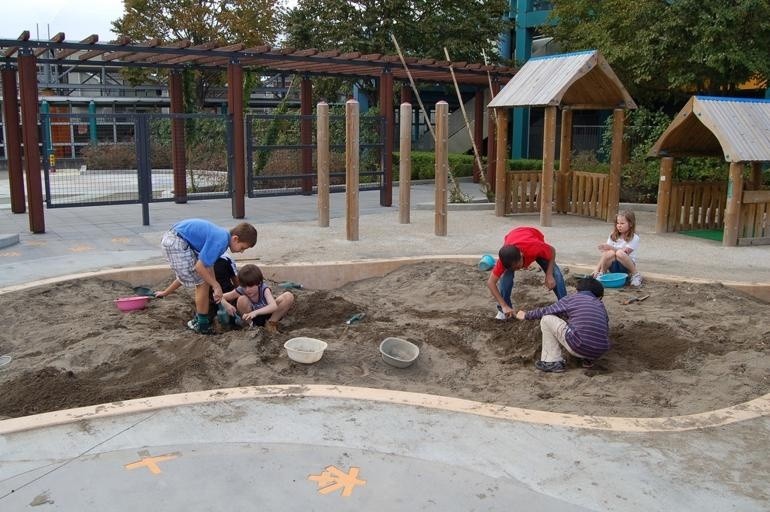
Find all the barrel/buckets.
[478,254,496,271]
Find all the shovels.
[623,293,650,305]
[232,311,249,328]
[346,312,366,325]
[574,273,593,279]
[133,286,163,299]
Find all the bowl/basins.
[596,272,629,291]
[283,336,329,364]
[380,337,420,367]
[113,295,149,312]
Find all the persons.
[153,218,295,335]
[516,277,611,373]
[593,208,643,287]
[487,226,568,320]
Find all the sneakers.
[496,311,507,318]
[187,318,216,335]
[581,359,593,368]
[535,360,566,373]
[630,273,643,286]
